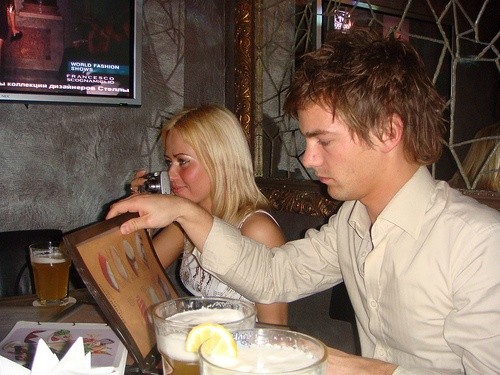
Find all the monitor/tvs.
[0,0,142,107]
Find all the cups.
[151,295,257,375]
[29,241,72,304]
[199,328,327,375]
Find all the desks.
[0,287,90,374]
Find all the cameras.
[138,170,170,195]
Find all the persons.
[130,105,288,327]
[105,26,500,375]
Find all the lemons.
[185,323,238,358]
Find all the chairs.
[0,229,66,296]
[249,185,348,326]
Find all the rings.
[130,186,137,193]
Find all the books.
[45,212,180,375]
